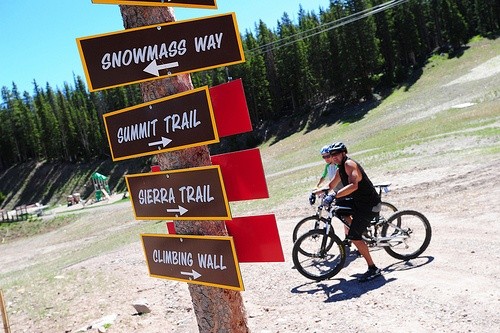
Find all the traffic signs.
[102,85,221,162]
[124,164,233,221]
[139,232,245,291]
[75,11,246,93]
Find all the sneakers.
[358,265,384,280]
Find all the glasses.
[322,154,331,159]
[331,152,342,156]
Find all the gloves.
[308,193,317,205]
[323,192,336,205]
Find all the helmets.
[328,142,348,154]
[320,145,332,154]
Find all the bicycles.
[292,183,402,258]
[292,191,432,281]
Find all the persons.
[312,145,351,248]
[309,141,381,281]
